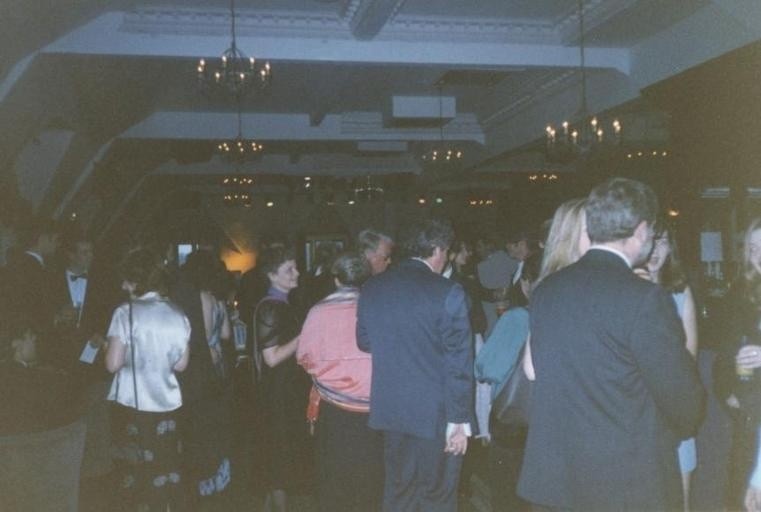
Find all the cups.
[496,299,508,319]
[735,335,754,382]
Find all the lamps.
[194,2,273,98]
[542,1,623,155]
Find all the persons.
[1,178,760,511]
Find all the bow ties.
[69,272,87,282]
